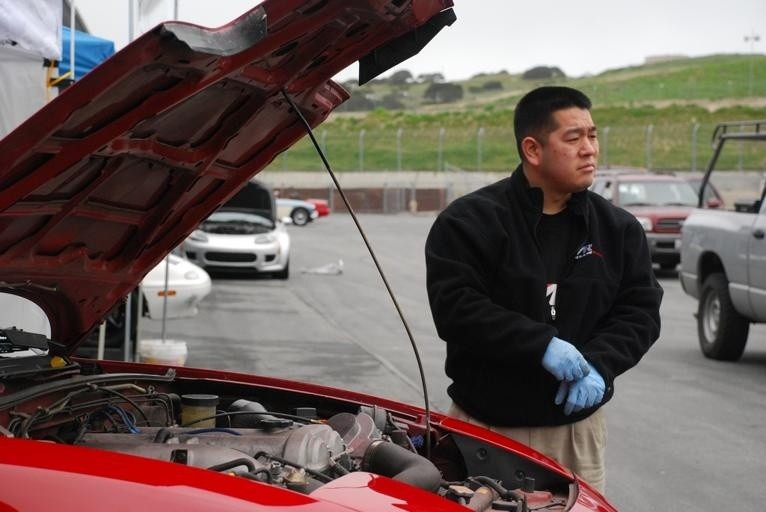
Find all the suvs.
[591,167,724,271]
[676,120,765,360]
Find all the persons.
[424,85,664,500]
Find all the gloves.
[555,361,606,415]
[541,335,591,382]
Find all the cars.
[141,180,329,318]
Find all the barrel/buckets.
[140,337,188,365]
